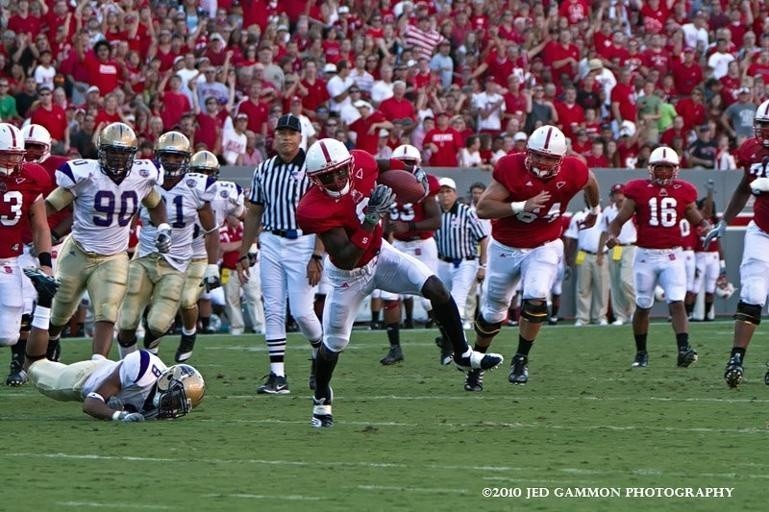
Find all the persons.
[0,0,769,430]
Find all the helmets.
[0,123,52,182]
[305,138,355,198]
[647,147,680,188]
[753,101,769,149]
[526,126,567,180]
[157,365,205,419]
[156,132,221,181]
[98,123,137,178]
[391,145,422,167]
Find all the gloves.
[199,277,218,293]
[118,411,145,421]
[154,231,172,254]
[365,185,397,224]
[417,170,430,203]
[702,221,726,249]
[750,178,769,195]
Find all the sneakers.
[311,385,334,428]
[381,346,403,364]
[257,372,289,394]
[678,347,697,367]
[144,328,161,355]
[508,355,528,383]
[632,350,648,367]
[46,339,61,363]
[7,361,29,386]
[175,331,196,362]
[23,266,60,294]
[310,350,317,389]
[434,336,503,392]
[724,364,743,388]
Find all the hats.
[438,177,456,190]
[275,116,302,132]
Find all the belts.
[272,228,314,237]
[438,252,474,262]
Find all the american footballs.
[378,169,426,203]
